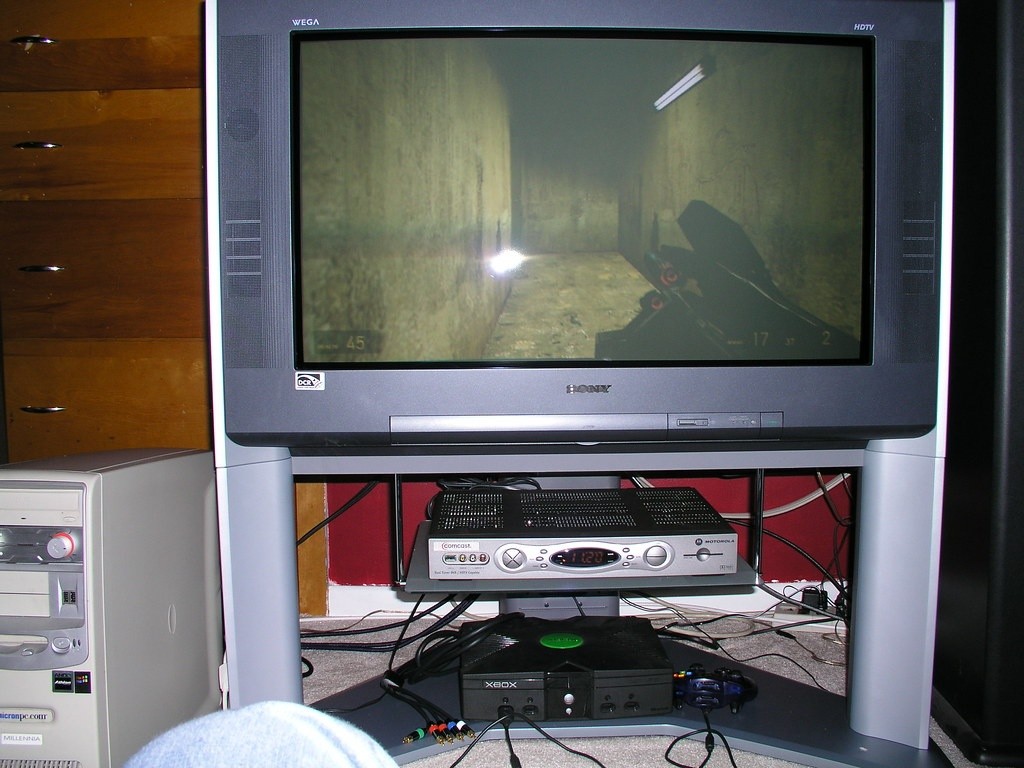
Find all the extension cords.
[772,603,849,637]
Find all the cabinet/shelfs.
[0,0,330,618]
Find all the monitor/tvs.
[205,0,946,460]
